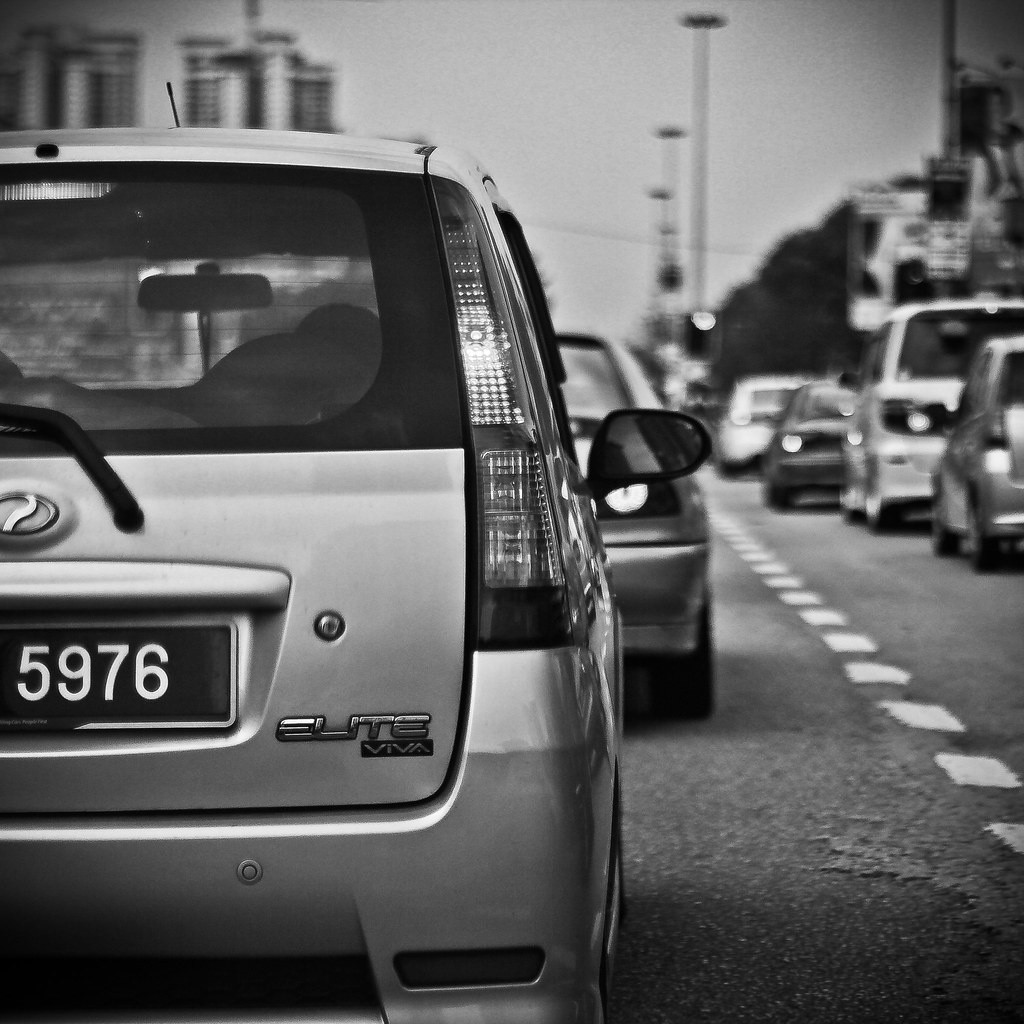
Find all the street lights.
[674,13,731,351]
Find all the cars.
[916,336,1024,570]
[718,374,817,476]
[556,328,713,723]
[764,383,851,507]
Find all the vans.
[838,296,1024,532]
[0,124,626,1024]
